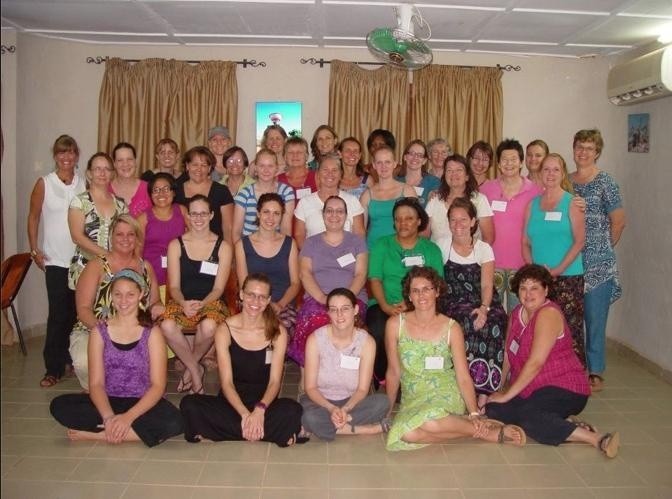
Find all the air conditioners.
[607,45,672,106]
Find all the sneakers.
[40,374,61,387]
[588,375,602,392]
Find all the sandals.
[472,419,526,447]
[567,414,620,459]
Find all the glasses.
[243,289,267,303]
[226,158,244,165]
[190,211,210,217]
[152,187,170,193]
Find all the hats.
[207,126,230,140]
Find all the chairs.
[0,252,33,355]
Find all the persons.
[180,272,311,448]
[49,264,187,448]
[385,265,527,451]
[299,285,393,440]
[479,264,620,459]
[25,122,626,415]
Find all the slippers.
[176,364,207,396]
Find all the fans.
[366,4,433,71]
